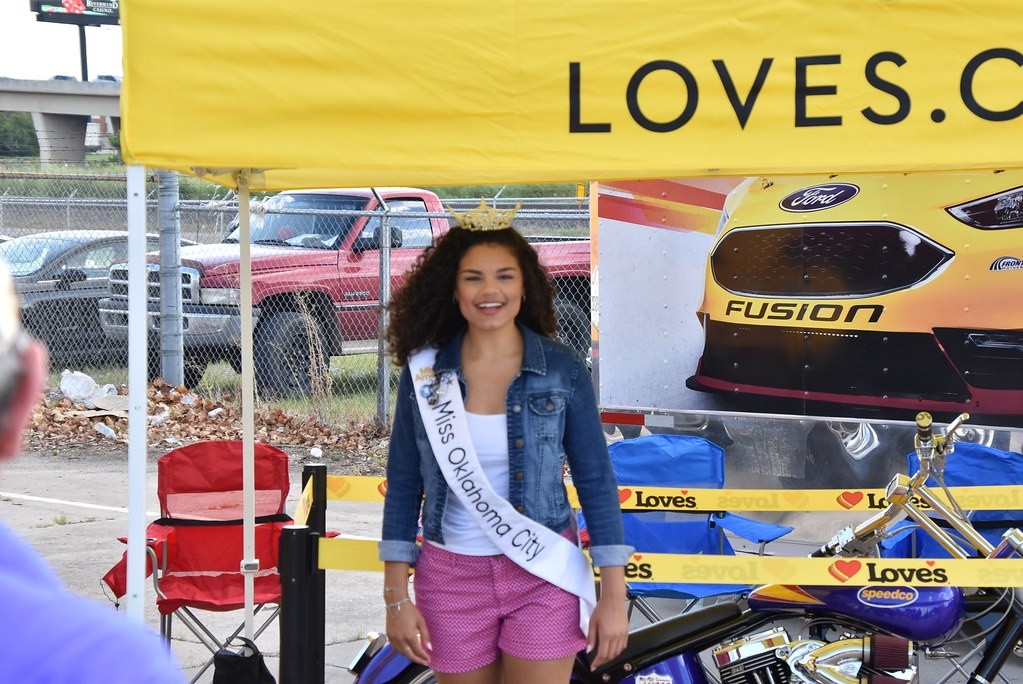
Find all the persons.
[0,259,185,683]
[378,196,634,684]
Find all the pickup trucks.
[98,188,589,396]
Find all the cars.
[684,171,1023,429]
[2,230,208,371]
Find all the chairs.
[100,438,295,683]
[371,226,403,248]
[871,441,1023,684]
[574,435,794,630]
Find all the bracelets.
[384,588,396,591]
[385,597,410,618]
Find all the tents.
[118,0,1023,630]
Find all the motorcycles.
[356,411,1023,684]
